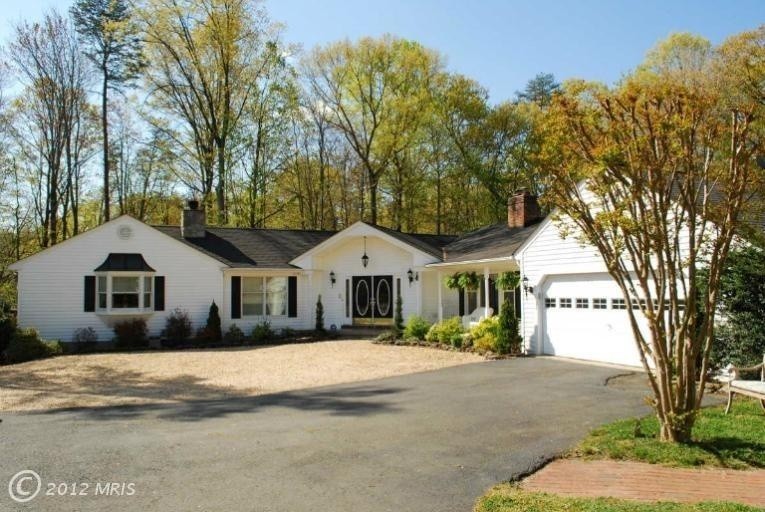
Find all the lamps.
[329,272,335,286]
[408,269,419,285]
[361,236,369,266]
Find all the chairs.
[724,363,765,414]
[462,306,494,329]
